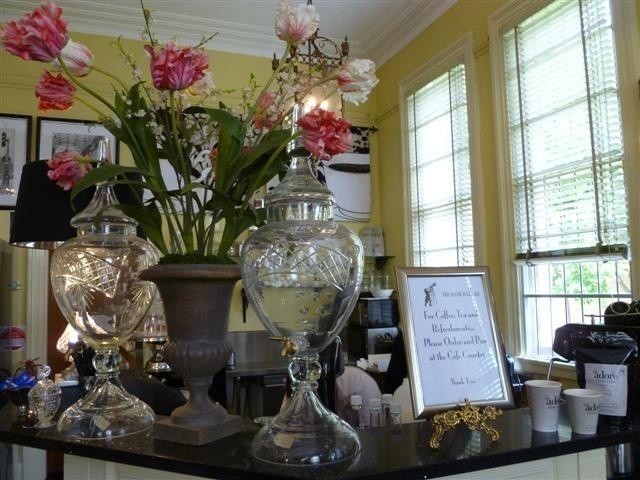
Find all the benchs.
[225,328,294,407]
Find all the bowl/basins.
[370,287,393,298]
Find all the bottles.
[348,393,403,429]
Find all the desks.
[216,360,334,419]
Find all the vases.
[135,262,253,447]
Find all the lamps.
[302,0,329,115]
[55,322,78,381]
[241,264,265,324]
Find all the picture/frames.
[35,115,119,166]
[1,113,32,210]
[393,265,516,420]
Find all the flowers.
[0,0,382,265]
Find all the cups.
[563,388,603,436]
[524,379,562,433]
